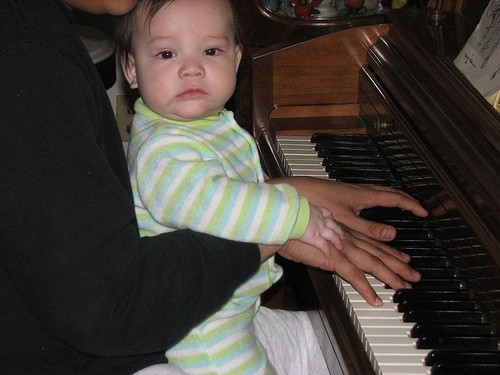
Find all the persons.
[0,0,428,375]
[117,0,348,374]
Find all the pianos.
[251,0,500,375]
